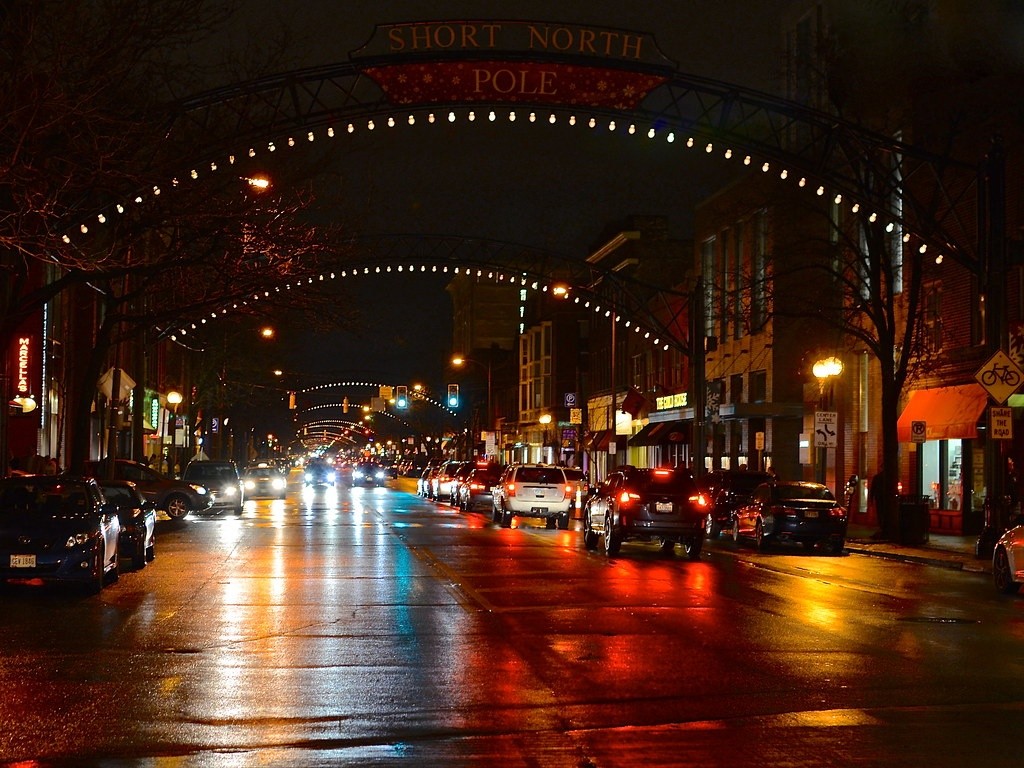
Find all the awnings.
[581,429,621,451]
[897,383,987,443]
[628,421,690,446]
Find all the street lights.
[538,414,552,464]
[324,357,492,453]
[104,177,282,479]
[551,285,617,469]
[812,356,845,485]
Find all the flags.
[621,389,646,417]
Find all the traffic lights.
[450,386,457,406]
[398,387,406,408]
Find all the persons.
[739,463,747,470]
[767,467,780,482]
[9,445,62,475]
[679,461,694,476]
[141,453,172,475]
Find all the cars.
[417,460,503,511]
[492,463,571,529]
[182,459,244,516]
[562,467,588,506]
[0,476,121,594]
[582,464,710,557]
[399,459,426,476]
[58,458,207,520]
[96,480,157,569]
[991,513,1024,594]
[703,469,775,539]
[304,455,398,488]
[244,456,300,499]
[731,481,846,553]
